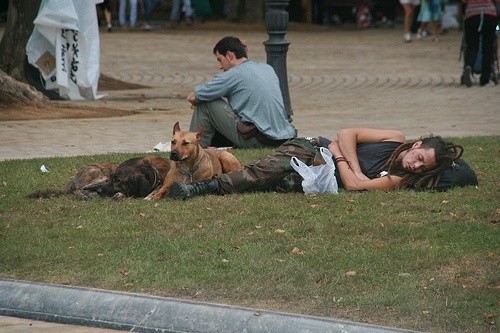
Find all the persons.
[169,0,193,30]
[140,0,158,31]
[186,36,296,150]
[167,127,464,201]
[96,0,115,32]
[400,0,444,44]
[119,0,139,33]
[461,0,498,88]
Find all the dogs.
[22,155,172,203]
[142,118,243,203]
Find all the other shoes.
[186,17,192,25]
[464,65,475,86]
[404,33,412,42]
[483,81,495,87]
[171,22,178,28]
[142,24,151,29]
[107,24,112,32]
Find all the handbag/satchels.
[290,146,338,196]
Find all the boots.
[172,178,220,201]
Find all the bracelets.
[335,157,346,162]
